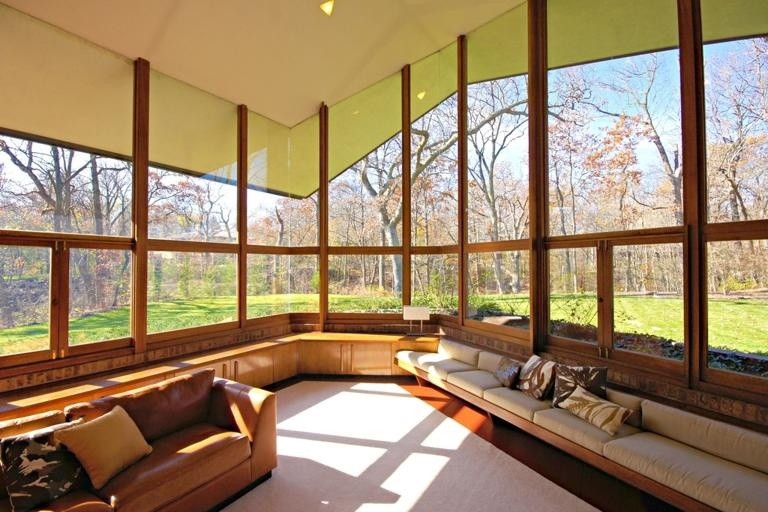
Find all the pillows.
[0,404,155,511]
[493,352,634,437]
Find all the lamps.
[402,305,430,336]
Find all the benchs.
[396,338,768,511]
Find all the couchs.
[0,367,278,511]
